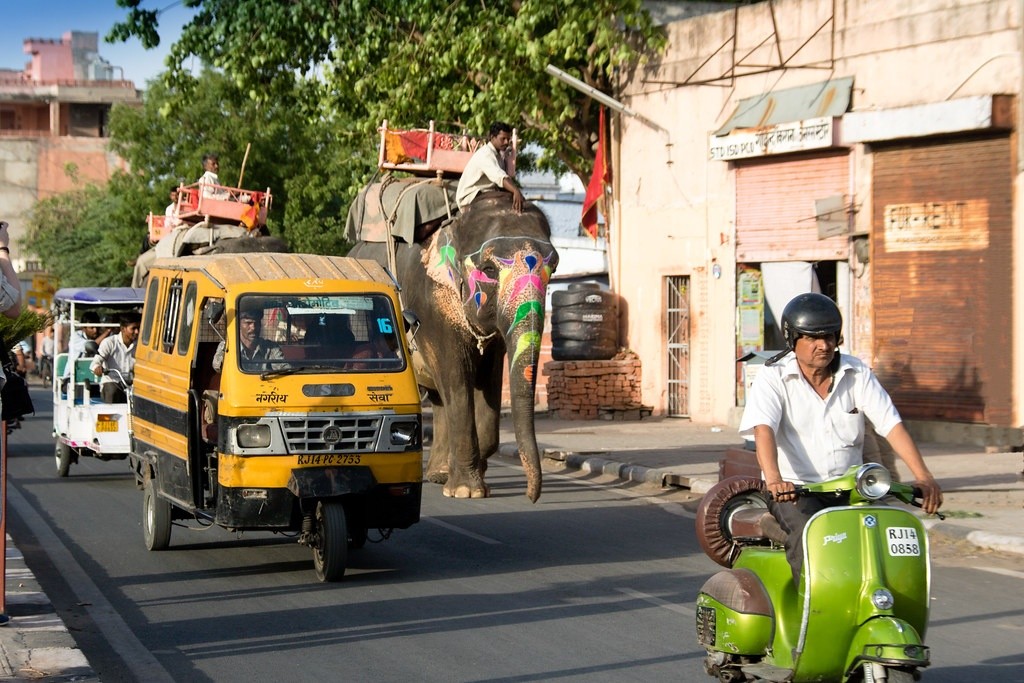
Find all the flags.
[580,105,610,243]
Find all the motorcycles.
[123,250,433,581]
[48,286,148,478]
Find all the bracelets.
[0,247,9,254]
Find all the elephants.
[188,233,290,254]
[346,185,560,504]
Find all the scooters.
[693,443,946,683]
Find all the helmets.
[782,293,842,351]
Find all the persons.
[164,185,189,232]
[455,121,525,216]
[88,312,143,403]
[291,309,355,365]
[738,293,943,591]
[198,153,254,205]
[212,299,292,374]
[61,310,120,393]
[344,314,411,371]
[40,328,54,377]
[0,220,32,430]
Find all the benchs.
[189,343,306,445]
[55,351,102,405]
